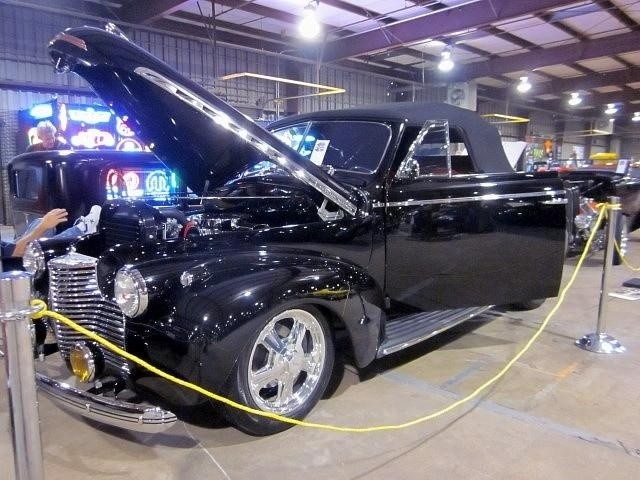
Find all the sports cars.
[533,155,640,266]
[32,21,574,439]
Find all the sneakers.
[80,205,102,234]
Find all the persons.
[0,204,103,345]
[24,120,71,152]
